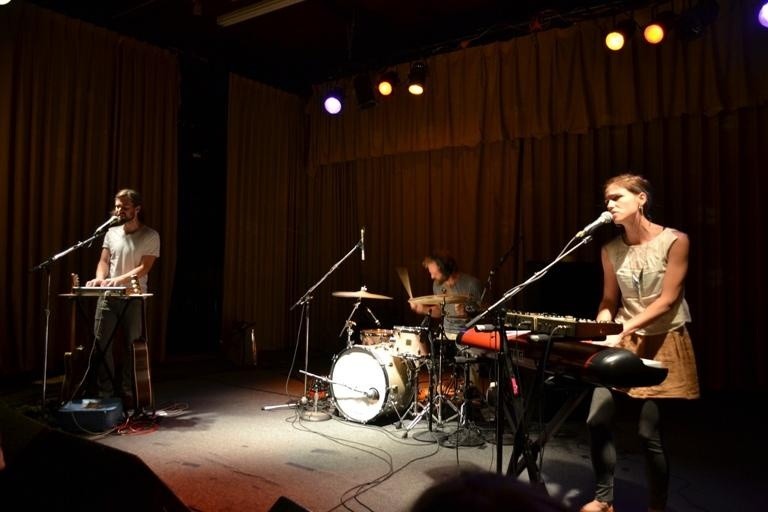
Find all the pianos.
[72,286,127,295]
[455,325,668,388]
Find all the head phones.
[429,252,453,276]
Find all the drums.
[393,325,432,359]
[360,329,392,345]
[328,345,415,425]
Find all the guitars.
[61,272,86,405]
[131,274,152,411]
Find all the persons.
[410,254,483,399]
[85,190,160,397]
[580,173,702,512]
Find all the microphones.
[359,225,366,262]
[92,215,119,235]
[577,211,615,240]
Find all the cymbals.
[408,296,470,304]
[333,291,393,300]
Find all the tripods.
[262,311,317,411]
[396,347,441,429]
[401,356,465,435]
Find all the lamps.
[601,0,717,53]
[318,47,427,118]
